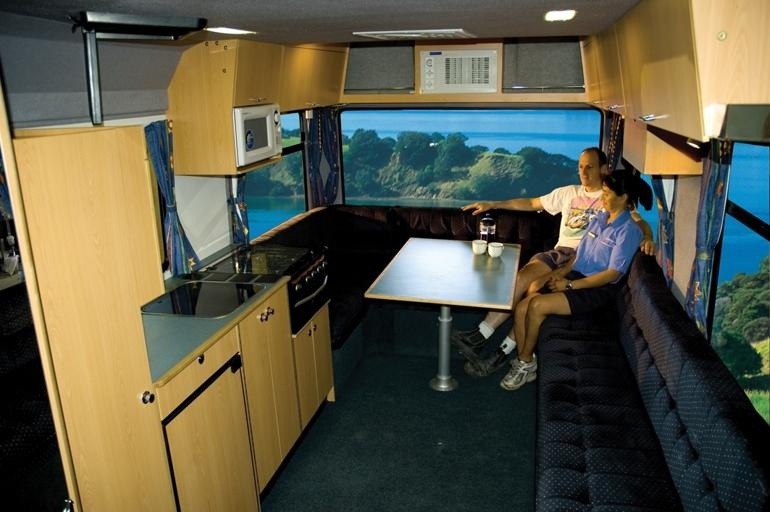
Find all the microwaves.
[233,103,282,167]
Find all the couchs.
[249,203,769,511]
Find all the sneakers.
[499,352,538,391]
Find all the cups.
[489,242,503,257]
[471,240,488,254]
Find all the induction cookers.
[141,283,265,318]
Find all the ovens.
[207,242,334,334]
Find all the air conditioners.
[419,50,497,95]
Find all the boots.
[448,327,491,366]
[466,346,513,377]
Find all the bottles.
[480,212,496,242]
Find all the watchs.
[565,280,574,292]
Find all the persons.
[451,146,658,379]
[497,168,654,392]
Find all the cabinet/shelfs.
[281,45,349,114]
[157,276,334,512]
[11,129,176,511]
[167,39,283,176]
[584,1,770,175]
